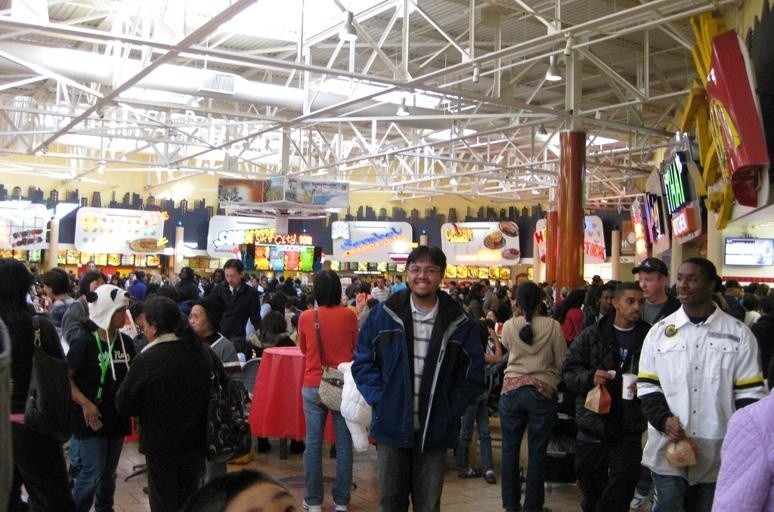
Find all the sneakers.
[630,492,650,509]
[506,503,552,512]
[302,498,321,512]
[483,467,496,483]
[334,501,348,512]
[459,468,482,477]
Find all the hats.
[192,294,225,317]
[86,284,130,331]
[632,258,668,276]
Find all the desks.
[249,346,358,488]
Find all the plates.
[483,222,520,259]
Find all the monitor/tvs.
[724,237,774,266]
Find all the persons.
[2,245,773,512]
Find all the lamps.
[396,98,410,116]
[545,55,561,81]
[338,11,358,42]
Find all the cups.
[621,373,639,401]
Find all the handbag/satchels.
[24,349,74,443]
[544,449,577,482]
[318,366,344,411]
[207,383,251,464]
